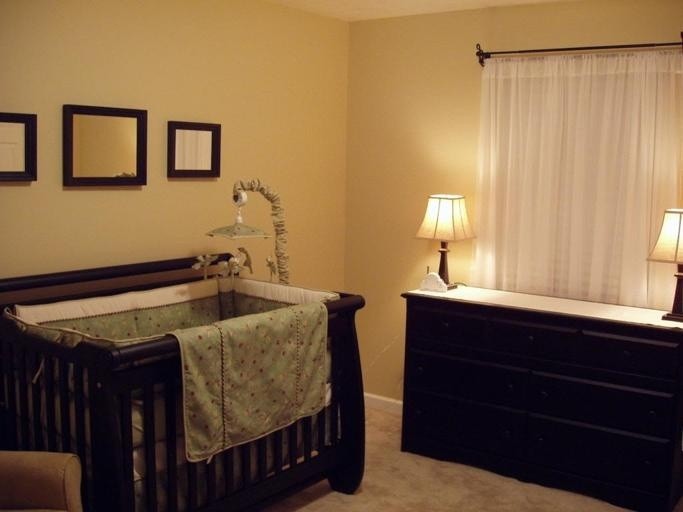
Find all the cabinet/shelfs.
[397,300,683,511]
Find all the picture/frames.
[60,103,148,186]
[0,112,40,180]
[167,120,220,178]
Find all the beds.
[5,273,365,511]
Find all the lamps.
[646,207,683,322]
[415,193,473,286]
